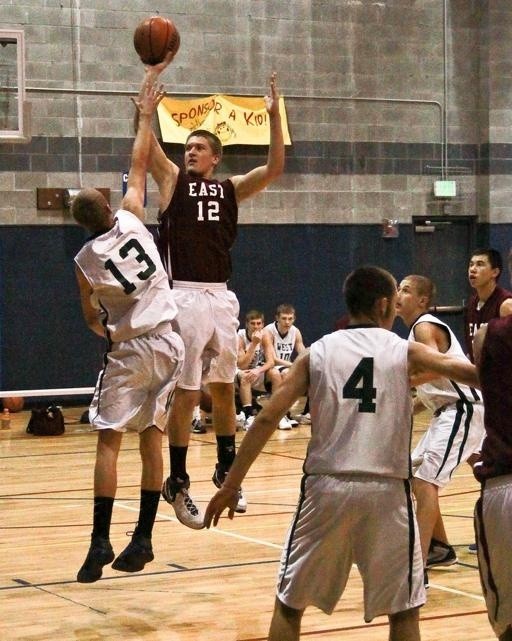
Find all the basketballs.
[3,397,23,413]
[134,15,180,65]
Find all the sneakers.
[111,533,154,572]
[278,412,312,430]
[425,540,457,565]
[161,473,206,530]
[192,415,207,433]
[77,543,115,584]
[211,462,247,513]
[234,410,256,432]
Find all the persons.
[125,51,288,532]
[459,247,511,555]
[393,274,489,591]
[198,264,480,641]
[263,302,313,427]
[68,79,187,585]
[192,348,214,435]
[469,243,511,641]
[233,309,296,433]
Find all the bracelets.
[222,482,243,492]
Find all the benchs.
[1,386,98,399]
